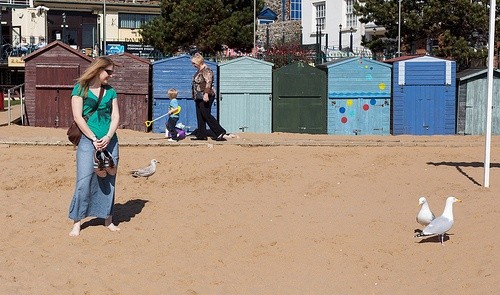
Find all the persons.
[36,36,47,48]
[16,38,28,51]
[166,88,179,142]
[189,52,227,141]
[68,56,120,236]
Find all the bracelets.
[105,134,111,140]
[92,135,97,142]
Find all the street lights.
[338,24,342,50]
[373,28,376,41]
[316,23,322,51]
[350,27,353,52]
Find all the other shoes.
[216,130,226,140]
[168,138,178,142]
[194,136,208,140]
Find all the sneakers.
[93,150,107,178]
[102,149,117,176]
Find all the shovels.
[145,106,181,127]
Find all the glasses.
[104,69,114,75]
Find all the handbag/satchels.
[66,84,105,148]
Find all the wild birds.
[412,195,462,244]
[416,197,436,227]
[126,159,160,181]
